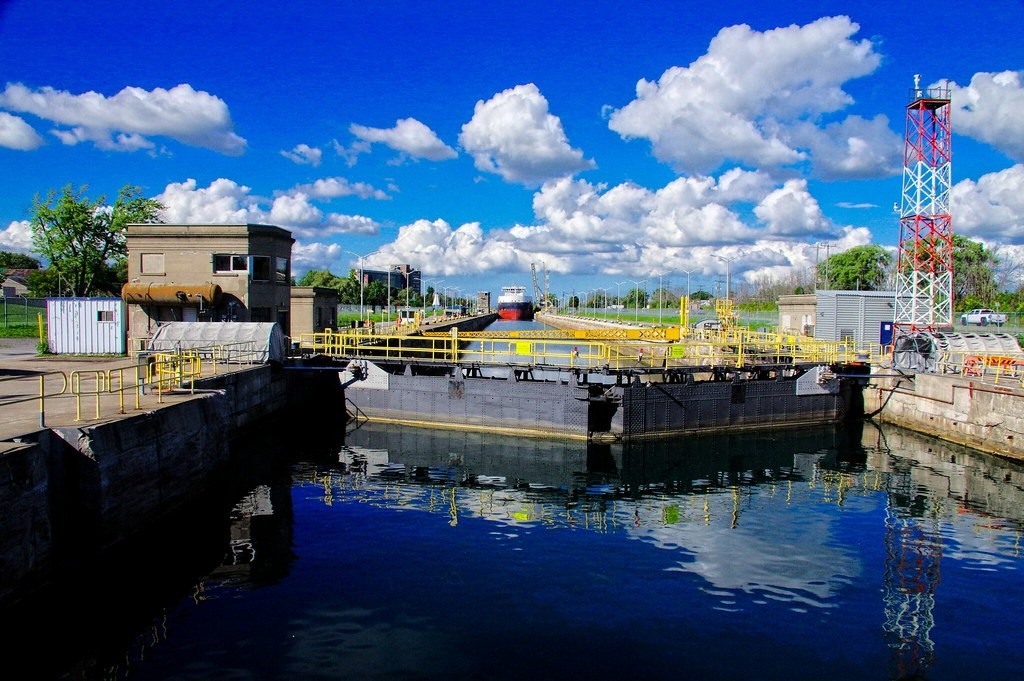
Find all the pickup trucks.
[961,309,1009,327]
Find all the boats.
[497,287,533,321]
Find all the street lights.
[592,288,600,319]
[348,251,380,321]
[551,291,590,317]
[375,265,400,326]
[601,287,612,318]
[654,271,672,324]
[611,281,627,320]
[711,254,742,309]
[414,277,478,320]
[674,267,703,310]
[395,267,417,324]
[629,280,649,324]
